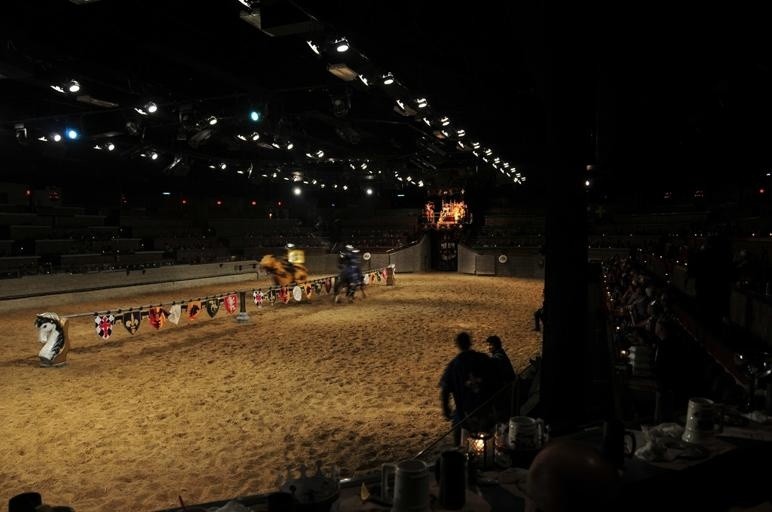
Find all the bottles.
[438,448,467,510]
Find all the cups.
[507,415,546,454]
[681,397,725,447]
[378,459,429,512]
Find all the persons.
[604,253,672,342]
[334,245,367,303]
[282,243,308,279]
[436,332,517,446]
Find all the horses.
[331,248,368,305]
[254,254,308,288]
